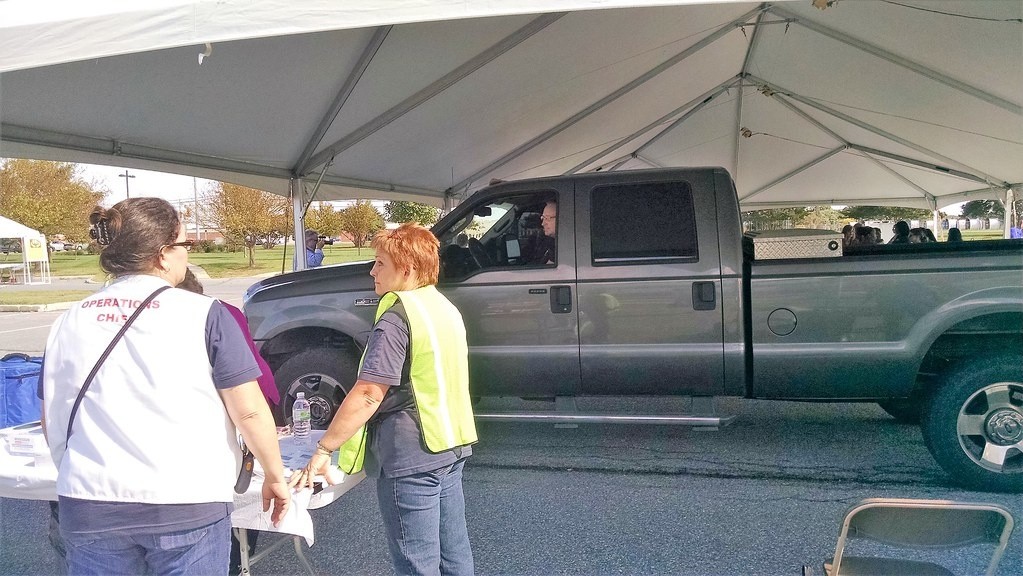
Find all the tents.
[0,216,43,282]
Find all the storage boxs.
[0,353,45,428]
[744,230,846,257]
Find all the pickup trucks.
[242,164,1023,493]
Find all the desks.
[1,420,371,576]
[0,264,24,284]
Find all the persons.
[842,221,963,246]
[36,197,291,576]
[284,221,479,576]
[305,229,325,269]
[541,201,556,264]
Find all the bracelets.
[316,440,333,453]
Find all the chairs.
[802,498,1015,576]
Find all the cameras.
[319,236,334,245]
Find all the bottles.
[292,392,311,446]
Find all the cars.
[317,235,334,245]
[1,242,21,254]
[245,232,280,245]
[47,240,83,252]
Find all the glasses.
[167,240,192,251]
[540,215,556,222]
